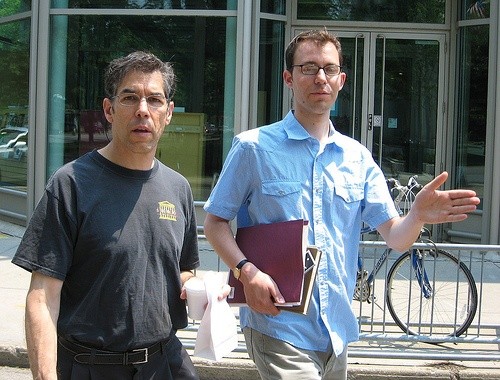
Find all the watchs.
[233,259,251,280]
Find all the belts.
[57,330,175,366]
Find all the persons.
[202,29,481,380]
[10,50,200,380]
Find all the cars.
[1,127,30,160]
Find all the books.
[224,219,310,306]
[242,248,322,315]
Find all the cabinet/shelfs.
[155,112,204,201]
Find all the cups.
[185,280,208,320]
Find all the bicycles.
[354,174,478,345]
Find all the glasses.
[111,92,167,109]
[292,62,342,76]
